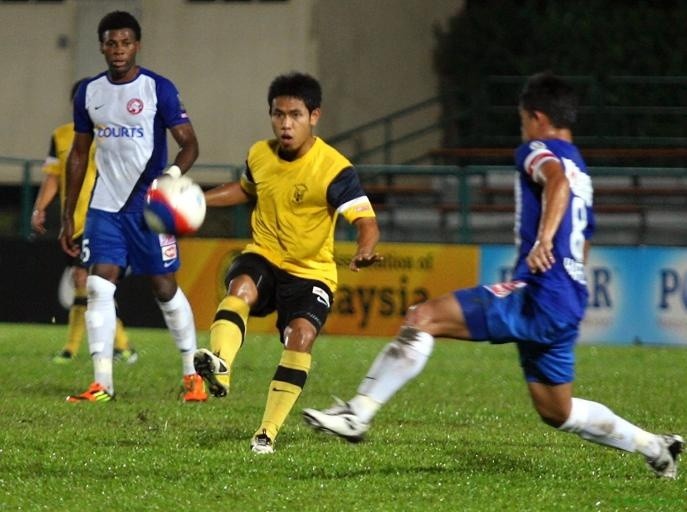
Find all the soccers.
[143,175,205,235]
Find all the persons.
[192,73,381,455]
[300,73,682,481]
[56,11,208,404]
[32,75,137,365]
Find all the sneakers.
[648,434,684,478]
[54,351,76,364]
[113,348,138,364]
[194,348,230,397]
[250,434,273,454]
[182,374,207,401]
[302,403,370,443]
[66,382,116,402]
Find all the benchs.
[358,141,687,244]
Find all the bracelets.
[164,165,182,179]
